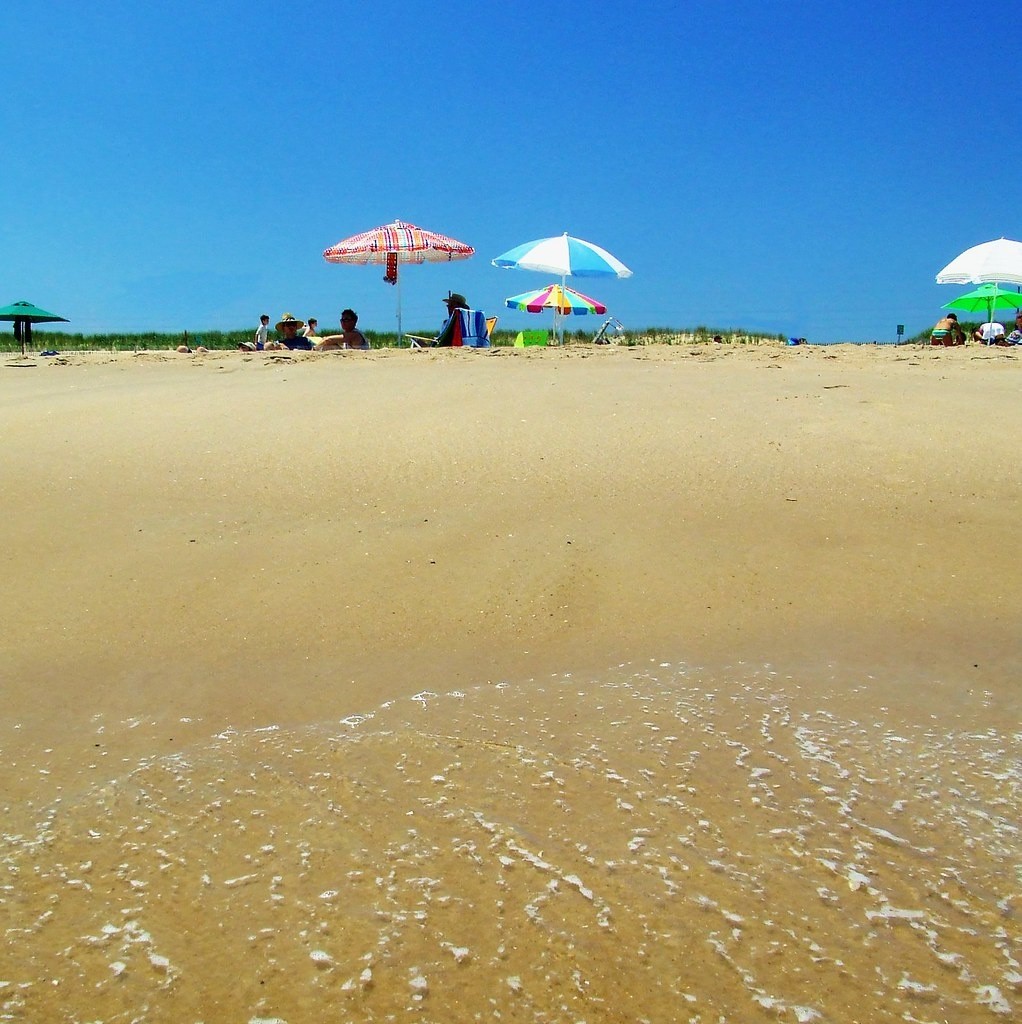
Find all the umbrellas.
[935,236,1022,346]
[506,283,607,340]
[324,219,475,349]
[490,232,633,346]
[940,284,1022,323]
[0,301,71,356]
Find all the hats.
[442,294,470,310]
[714,336,722,339]
[238,342,256,351]
[275,312,304,331]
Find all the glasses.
[340,319,354,322]
[447,303,455,308]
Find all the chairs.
[405,310,497,348]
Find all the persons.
[442,294,470,333]
[931,313,967,348]
[974,314,1022,347]
[255,309,369,351]
